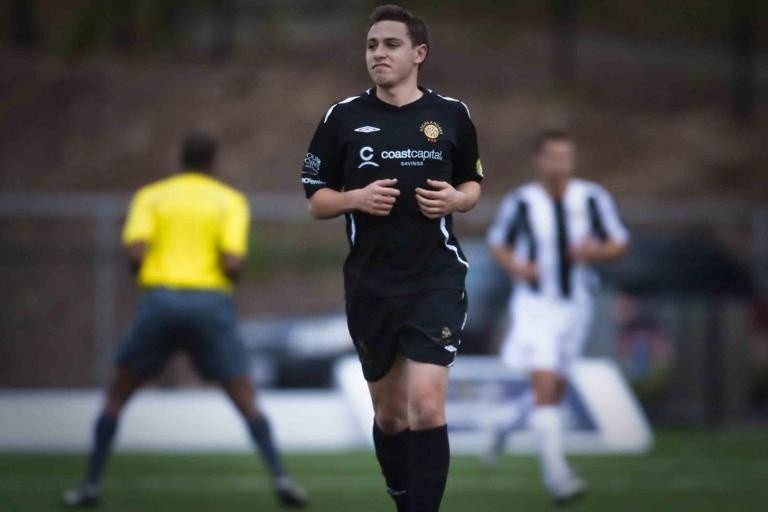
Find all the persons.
[62,131,308,510]
[484,131,633,500]
[301,6,486,512]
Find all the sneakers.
[550,479,586,505]
[62,489,96,510]
[275,480,306,508]
